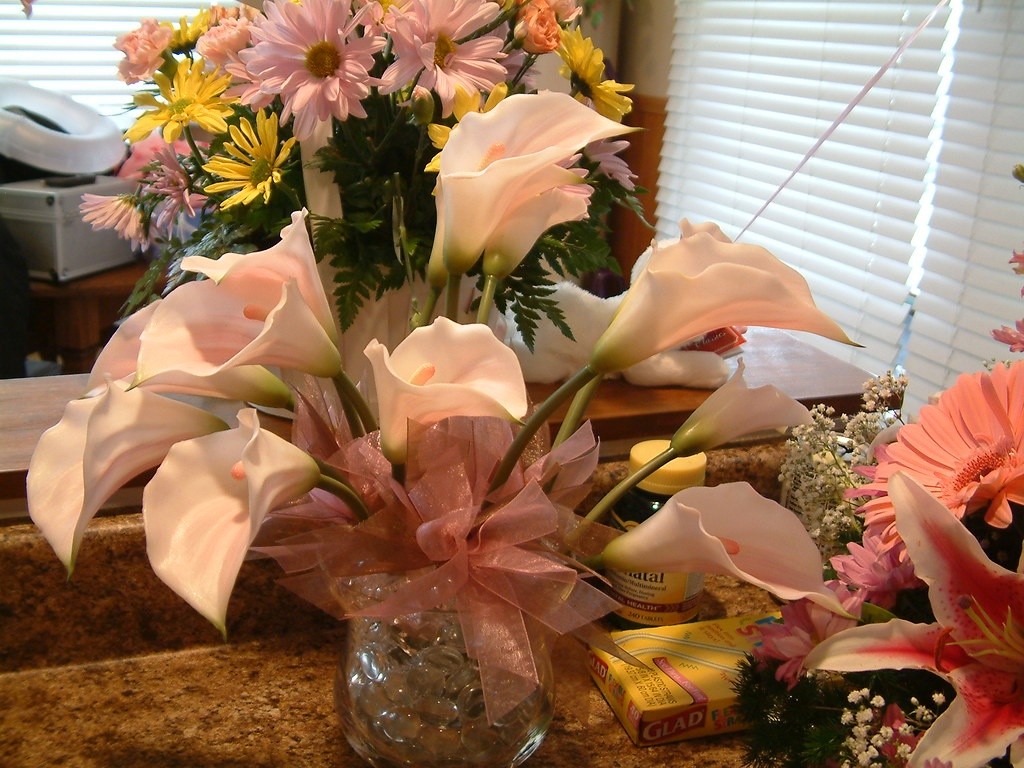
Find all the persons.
[0,218,76,377]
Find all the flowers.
[24,0,1024,768]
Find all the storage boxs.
[585,612,782,748]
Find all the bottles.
[610,439,711,628]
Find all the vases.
[333,556,555,768]
